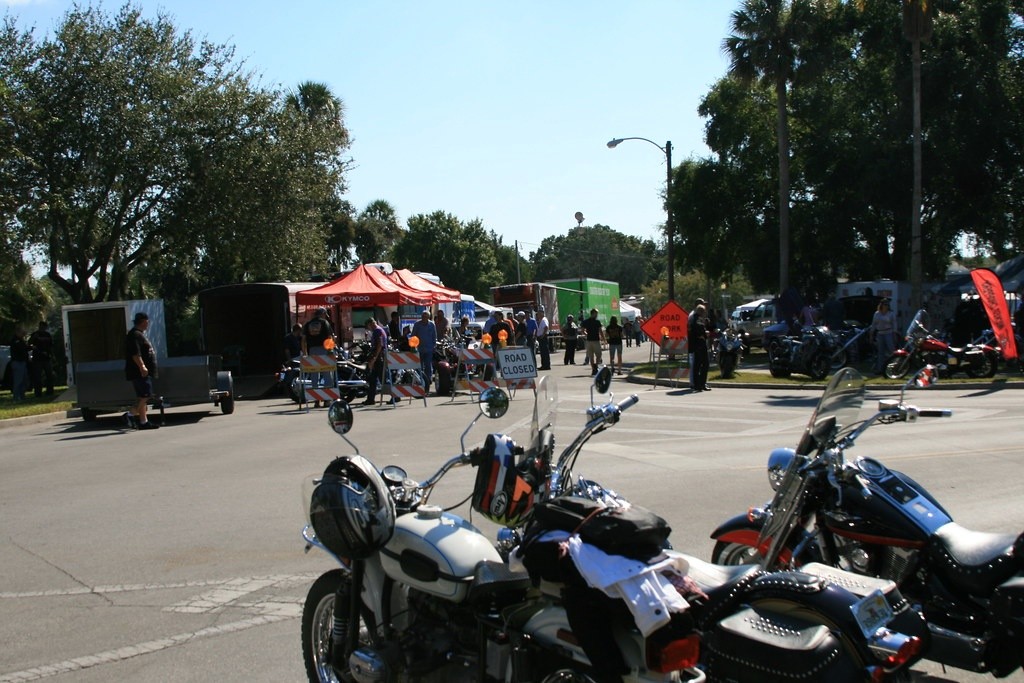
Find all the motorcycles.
[489,364,930,683]
[883,308,1003,381]
[274,327,476,406]
[301,387,707,683]
[710,361,1024,680]
[828,319,878,371]
[768,318,838,381]
[713,327,750,378]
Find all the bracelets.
[140,365,145,368]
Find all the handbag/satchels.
[537,491,671,559]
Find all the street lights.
[606,136,676,363]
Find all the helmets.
[310,455,397,561]
[472,433,552,529]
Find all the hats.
[131,312,148,321]
[316,307,329,317]
[695,298,708,304]
[293,323,303,329]
[364,318,374,327]
[515,311,525,316]
[567,315,574,321]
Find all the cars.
[735,300,779,357]
[762,319,794,362]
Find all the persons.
[302,309,332,408]
[871,298,896,373]
[624,315,647,347]
[27,321,54,397]
[792,290,843,338]
[362,317,401,405]
[282,322,303,361]
[687,297,730,392]
[580,309,605,376]
[389,310,538,394]
[605,316,623,375]
[9,326,28,402]
[561,314,578,365]
[122,311,159,430]
[536,309,551,370]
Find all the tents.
[386,268,463,320]
[295,263,432,347]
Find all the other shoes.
[324,401,330,406]
[611,369,614,375]
[592,371,596,375]
[538,367,550,370]
[592,364,598,373]
[362,400,375,404]
[704,387,711,391]
[123,413,138,429]
[618,372,623,375]
[314,403,322,408]
[386,397,401,404]
[138,421,158,429]
